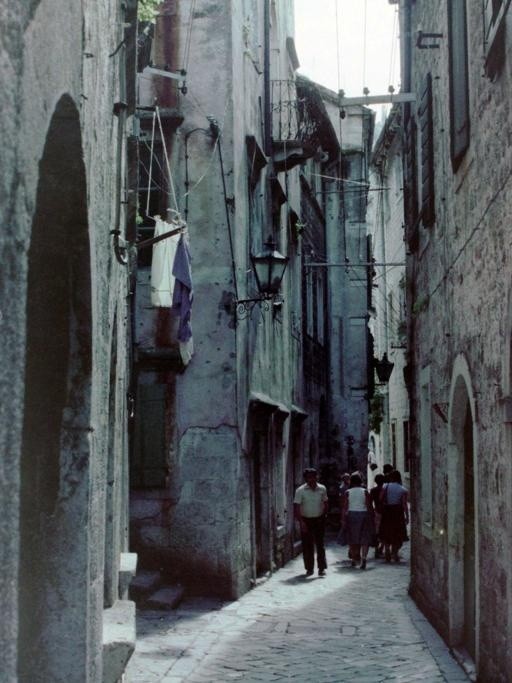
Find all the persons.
[337,446,410,569]
[291,466,330,576]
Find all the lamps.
[371,351,398,385]
[235,233,291,318]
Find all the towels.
[170,228,196,346]
